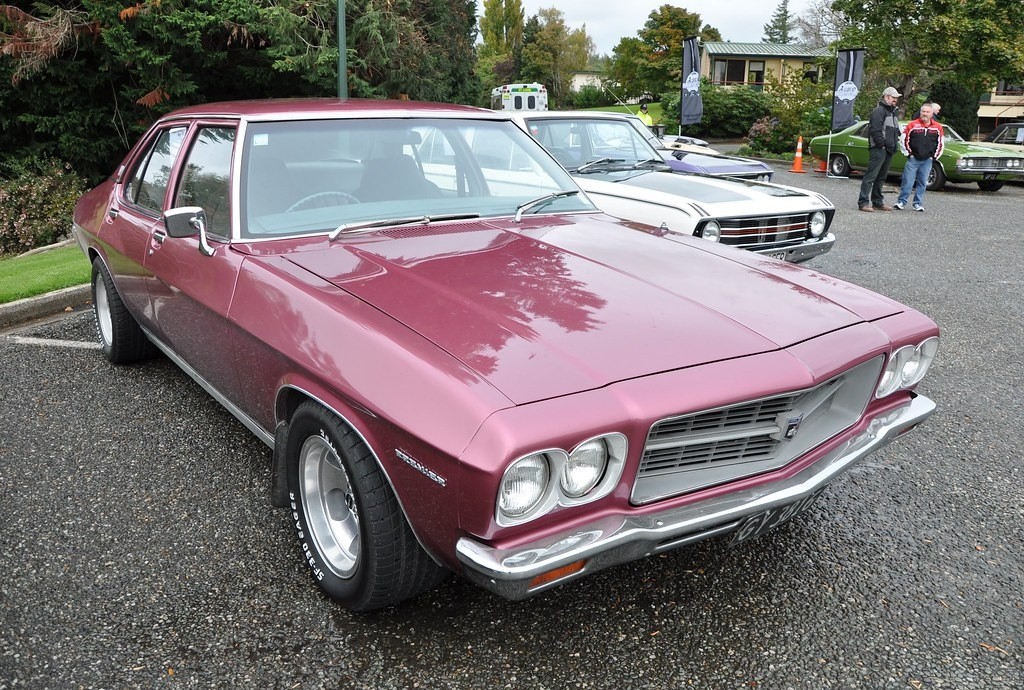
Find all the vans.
[491,82,548,109]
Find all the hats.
[881,86,903,97]
[640,104,648,110]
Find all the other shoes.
[859,204,874,212]
[872,204,893,211]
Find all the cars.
[71,97,941,614]
[808,119,1024,193]
[980,122,1024,145]
[410,109,837,265]
[566,122,774,184]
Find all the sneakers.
[893,202,905,209]
[913,204,924,211]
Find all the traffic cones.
[788,135,808,172]
[813,160,827,172]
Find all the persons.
[857,86,903,212]
[893,100,945,212]
[636,104,653,132]
